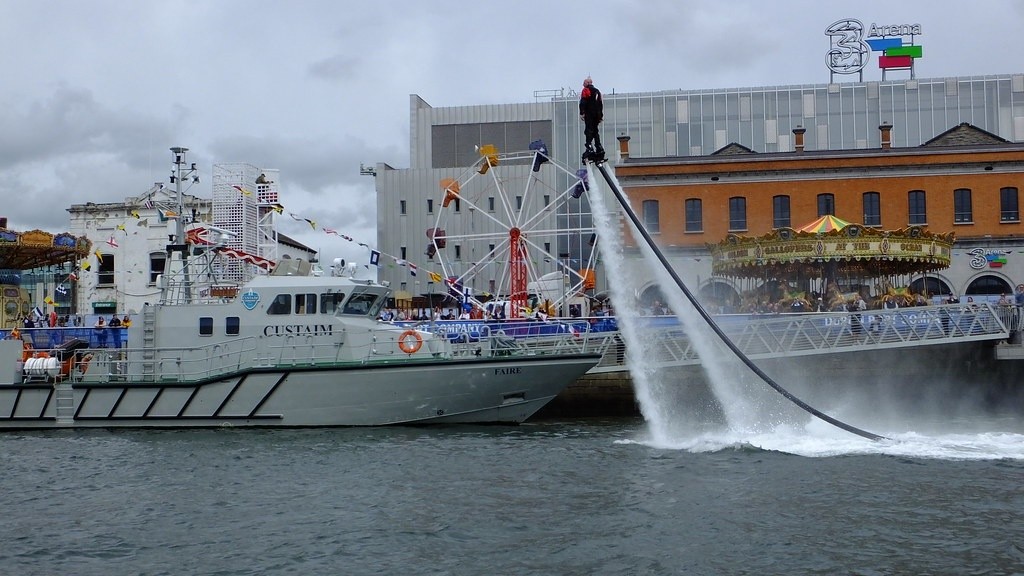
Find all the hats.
[1000,293,1004,296]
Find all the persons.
[998,293,1010,334]
[845,295,928,343]
[791,291,824,312]
[382,304,508,322]
[95,312,131,349]
[940,292,976,335]
[525,302,548,322]
[636,300,678,317]
[570,303,604,324]
[255,174,272,201]
[578,79,605,153]
[707,299,776,314]
[28,313,85,343]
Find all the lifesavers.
[398,331,423,354]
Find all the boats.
[0,148,621,434]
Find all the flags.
[410,262,417,276]
[370,249,381,266]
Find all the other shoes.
[114,346,119,348]
[119,346,122,348]
[97,345,102,348]
[103,346,109,348]
